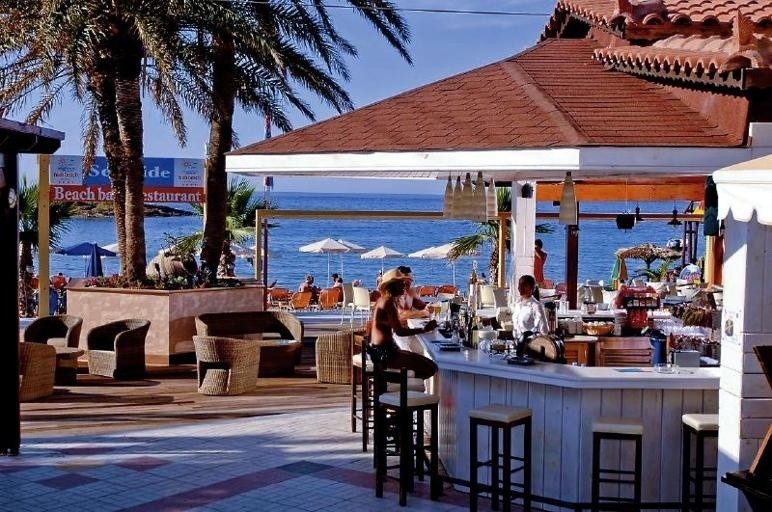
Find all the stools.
[681,414,719,512]
[468,403,532,512]
[591,417,643,512]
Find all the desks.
[258,340,302,378]
[55,347,85,382]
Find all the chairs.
[24,315,83,347]
[351,331,440,506]
[266,280,644,328]
[19,342,57,402]
[315,328,369,384]
[193,336,260,395]
[31,274,72,318]
[87,319,151,380]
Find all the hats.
[375,268,414,293]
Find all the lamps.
[635,201,644,224]
[667,200,683,229]
[442,171,499,223]
[616,179,634,229]
[559,171,578,226]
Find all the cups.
[648,310,713,341]
[428,304,442,325]
[613,313,626,336]
[557,299,583,334]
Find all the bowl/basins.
[582,324,612,336]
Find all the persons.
[394,265,434,329]
[679,257,700,279]
[331,273,343,288]
[298,273,321,305]
[509,274,548,343]
[534,239,548,288]
[376,270,385,285]
[370,268,440,380]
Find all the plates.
[436,342,463,351]
[507,357,534,365]
[654,363,681,374]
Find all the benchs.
[195,310,304,366]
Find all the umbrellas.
[361,245,407,277]
[298,237,350,287]
[426,242,476,285]
[610,255,628,281]
[84,243,103,278]
[335,238,367,279]
[406,246,448,282]
[54,241,119,276]
[239,245,270,259]
[102,243,121,256]
[161,238,257,256]
